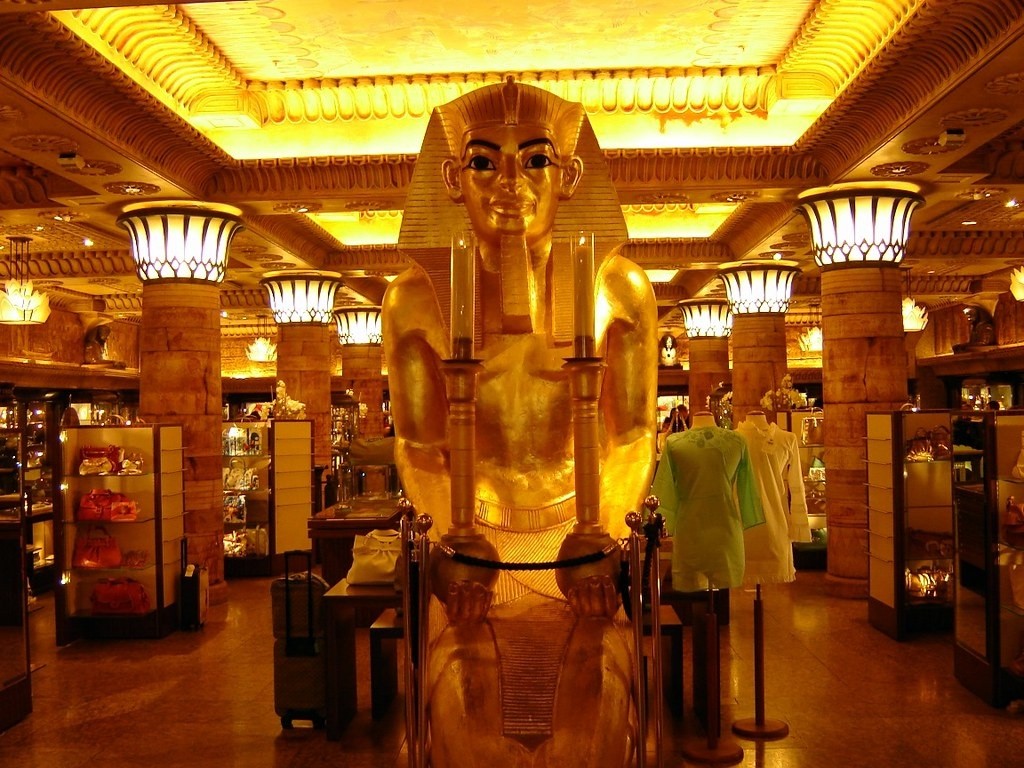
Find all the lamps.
[116,200,245,283]
[677,298,734,338]
[332,306,383,344]
[798,304,823,353]
[259,269,343,324]
[1009,265,1024,303]
[245,315,278,362]
[901,267,929,333]
[796,182,927,267]
[717,259,803,315]
[0,237,51,325]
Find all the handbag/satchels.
[223,525,269,559]
[223,493,246,524]
[346,529,401,586]
[1004,498,1024,547]
[905,425,951,462]
[73,526,121,569]
[93,576,148,614]
[77,488,129,520]
[270,571,329,639]
[905,566,948,598]
[223,457,259,491]
[222,420,261,455]
[79,457,115,476]
[801,407,824,445]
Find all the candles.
[452,241,473,339]
[574,236,594,337]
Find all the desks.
[307,492,730,742]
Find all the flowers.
[759,374,806,411]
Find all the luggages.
[179,537,209,629]
[273,550,329,730]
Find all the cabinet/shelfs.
[0,397,57,736]
[950,409,1024,708]
[861,410,953,643]
[775,409,827,571]
[222,419,314,579]
[58,423,189,641]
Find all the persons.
[84,324,127,369]
[650,412,812,592]
[382,83,659,768]
[660,337,677,366]
[963,308,996,345]
